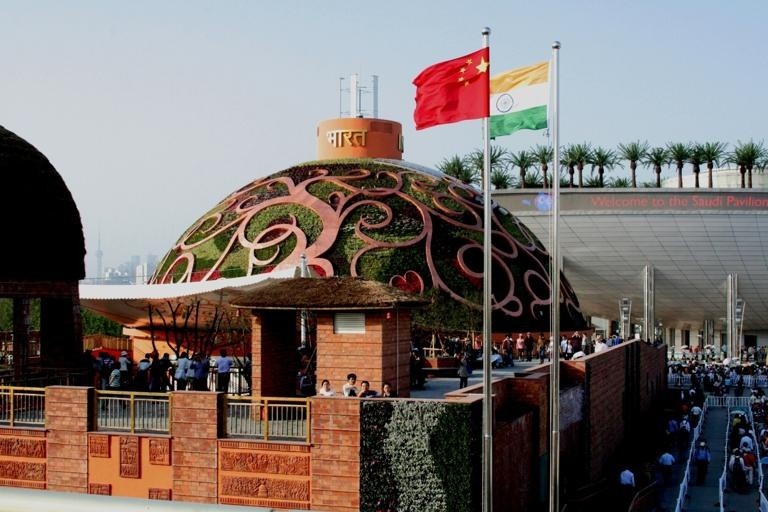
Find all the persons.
[241,352,252,395]
[3,350,14,366]
[359,381,379,397]
[652,336,767,504]
[317,380,337,397]
[84,345,211,413]
[382,381,398,397]
[212,348,233,395]
[620,465,636,494]
[342,373,359,397]
[297,353,311,389]
[408,329,636,390]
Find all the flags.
[411,46,490,130]
[481,60,550,141]
